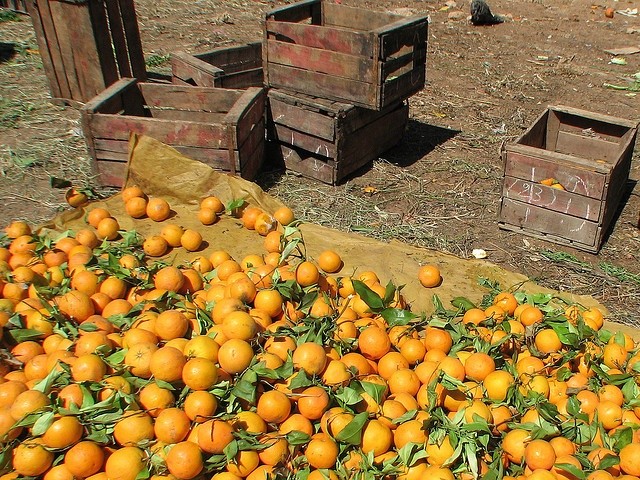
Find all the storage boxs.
[493,102,639,255]
[77,76,266,190]
[266,88,410,188]
[258,0,434,114]
[26,0,147,110]
[165,39,261,89]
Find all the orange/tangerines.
[417,266,441,287]
[295,283,640,480]
[541,178,564,192]
[0,185,379,480]
